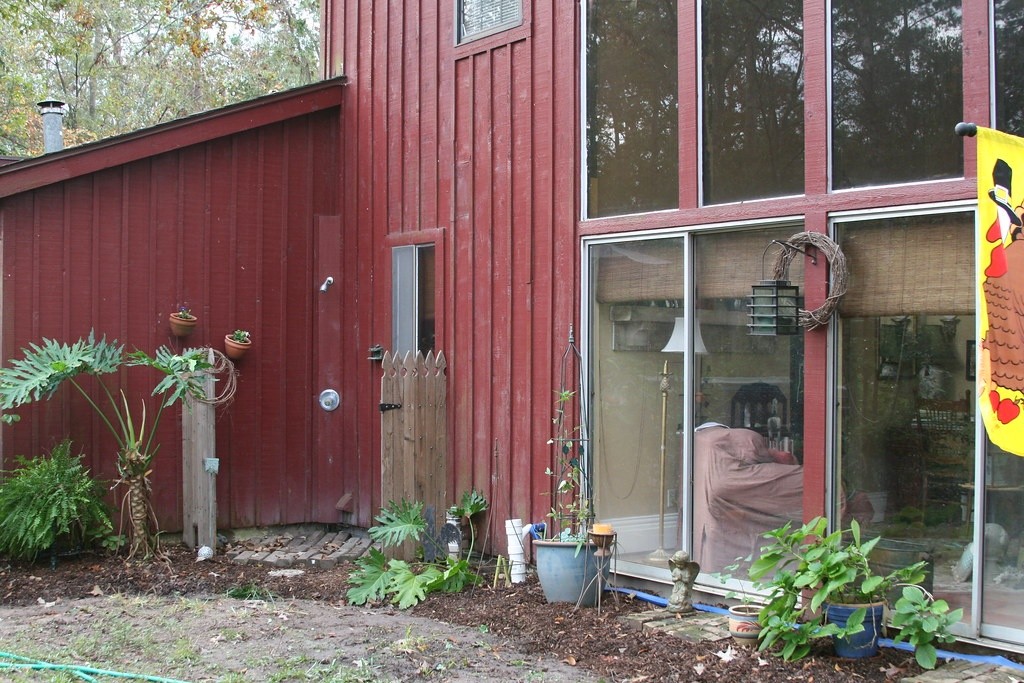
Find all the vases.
[225,329,252,359]
[169,312,198,337]
[532,389,610,605]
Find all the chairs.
[911,387,1024,542]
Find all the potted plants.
[749,515,964,670]
[711,553,769,647]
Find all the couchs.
[691,425,874,587]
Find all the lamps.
[745,239,817,336]
[913,324,956,399]
[661,318,709,356]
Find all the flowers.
[178,307,193,319]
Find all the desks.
[886,425,1024,527]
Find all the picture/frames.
[876,316,917,379]
[966,339,976,380]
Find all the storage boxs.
[959,454,1008,523]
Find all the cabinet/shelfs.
[732,382,794,439]
